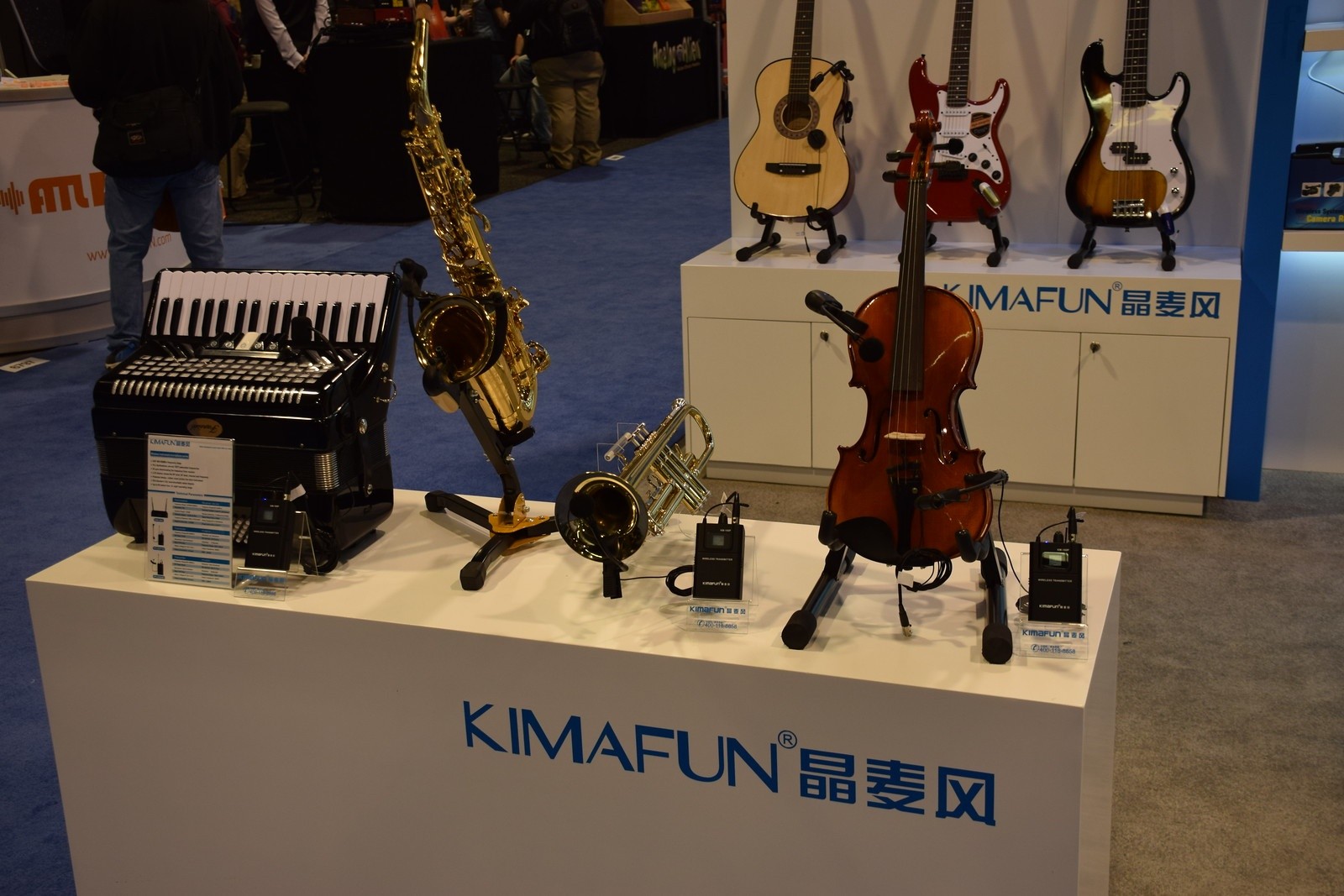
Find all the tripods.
[398,255,558,592]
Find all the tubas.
[552,397,717,564]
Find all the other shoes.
[539,161,560,170]
[104,347,135,369]
[499,67,517,84]
[223,190,259,202]
[274,182,310,194]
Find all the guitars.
[891,0,1013,224]
[1061,0,1198,230]
[731,0,857,222]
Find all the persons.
[438,0,604,176]
[240,0,332,184]
[69,0,244,369]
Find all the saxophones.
[397,16,554,437]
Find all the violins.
[825,103,995,570]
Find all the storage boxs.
[335,6,416,25]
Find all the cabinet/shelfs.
[25,490,1121,896]
[1225,0,1344,504]
[678,238,1242,515]
[599,23,725,136]
[313,41,499,224]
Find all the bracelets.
[514,53,522,56]
[456,13,464,21]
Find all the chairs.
[227,46,318,212]
[488,40,549,162]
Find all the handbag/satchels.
[92,84,198,177]
